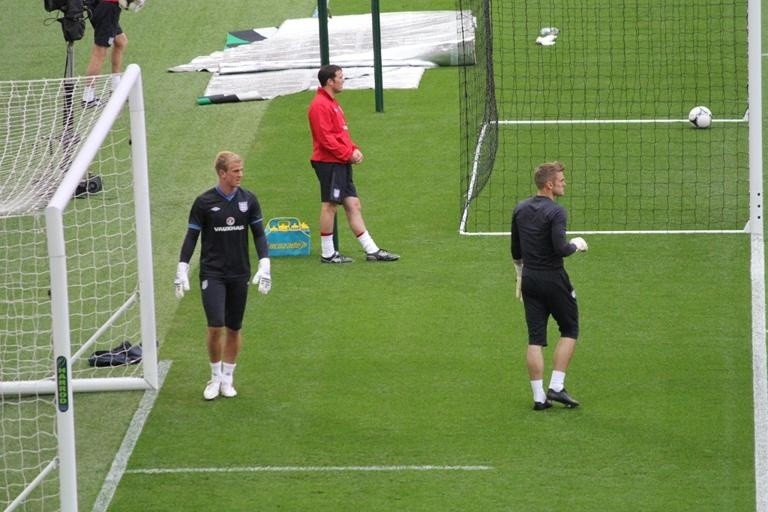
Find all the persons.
[307,65,402,264]
[509,160,589,412]
[175,150,271,399]
[81,0,145,108]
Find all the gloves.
[174,261,190,301]
[251,257,272,294]
[513,263,524,302]
[569,237,588,252]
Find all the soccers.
[689,106,711,127]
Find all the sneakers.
[364,249,399,261]
[546,387,580,406]
[221,376,238,397]
[203,377,221,400]
[320,250,354,264]
[534,400,553,410]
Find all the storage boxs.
[264,217,312,258]
